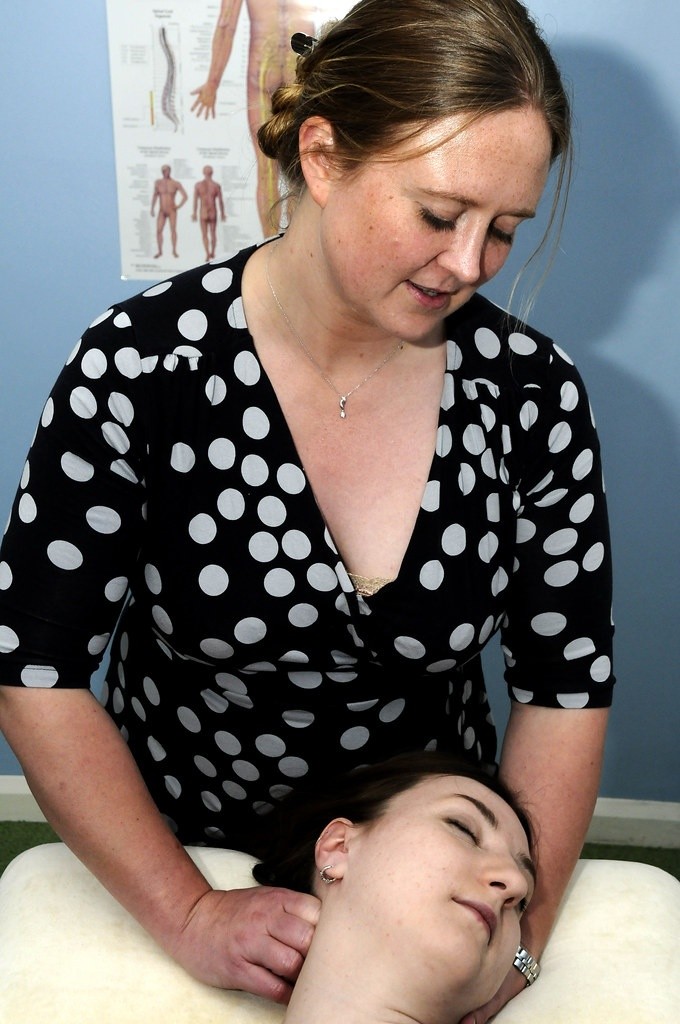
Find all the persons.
[0,0,617,1024]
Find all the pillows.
[0,838,680,1024]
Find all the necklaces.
[267,239,407,418]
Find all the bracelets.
[513,945,541,986]
